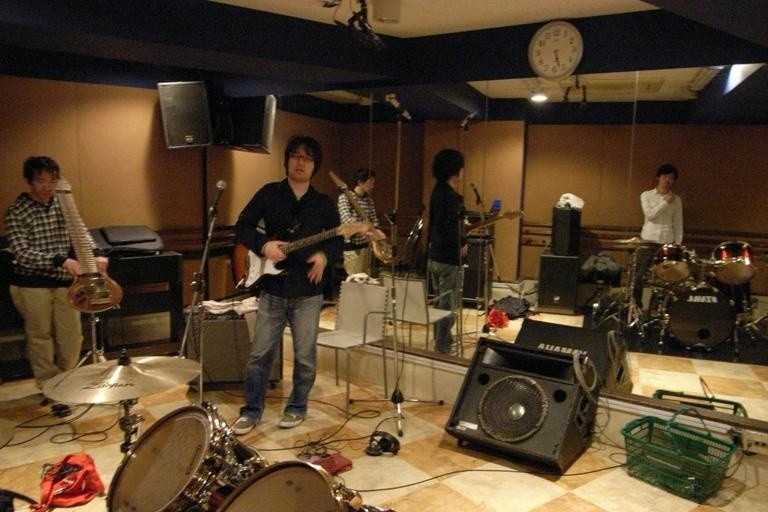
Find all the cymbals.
[615,237,655,244]
[41,356,203,405]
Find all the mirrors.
[200,63,768,434]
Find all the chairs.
[313,282,394,399]
[380,273,458,351]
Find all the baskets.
[620,407,737,504]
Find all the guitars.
[464,209,527,240]
[232,223,369,292]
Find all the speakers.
[537,246,580,315]
[514,317,634,394]
[551,206,582,256]
[444,336,602,476]
[156,80,215,150]
[184,314,282,386]
[427,236,495,305]
[215,93,278,155]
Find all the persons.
[633,164,686,309]
[423,147,469,356]
[228,133,341,435]
[336,167,380,279]
[5,154,108,417]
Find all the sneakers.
[230,415,255,435]
[280,413,303,427]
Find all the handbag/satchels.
[31,453,104,512]
[488,295,535,319]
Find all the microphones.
[385,93,413,121]
[352,11,386,52]
[469,182,480,205]
[460,111,480,128]
[206,179,228,218]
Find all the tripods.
[349,209,444,437]
[589,250,644,339]
[72,315,107,368]
[600,253,644,321]
[451,200,514,344]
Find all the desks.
[99,251,185,348]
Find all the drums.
[106,400,270,512]
[713,240,759,285]
[669,284,737,348]
[213,460,396,512]
[655,244,691,282]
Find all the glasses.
[290,153,315,162]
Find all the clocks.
[526,22,586,83]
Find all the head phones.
[365,431,400,456]
